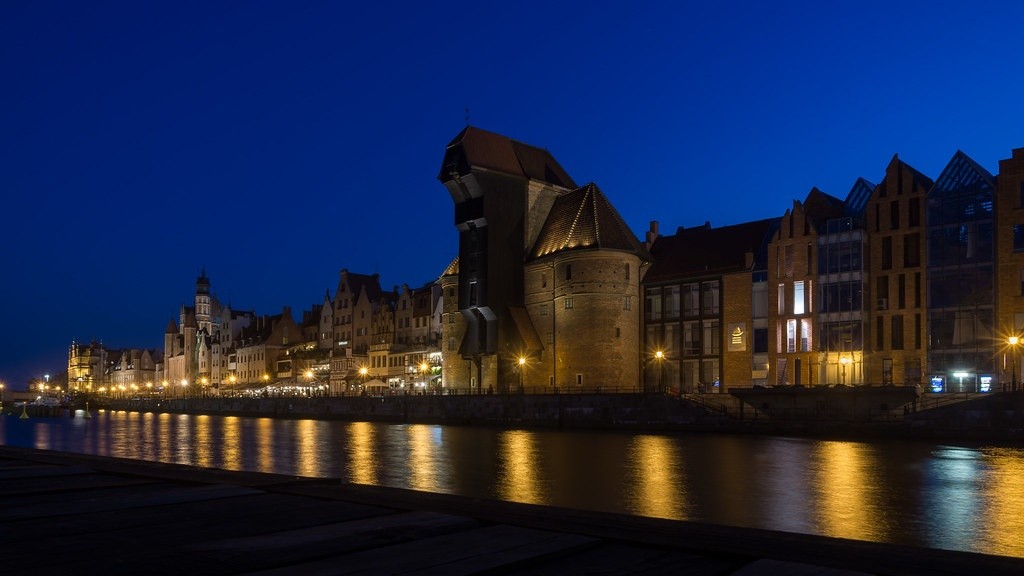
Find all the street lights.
[202,378,206,398]
[182,379,188,399]
[655,351,662,393]
[1007,335,1019,392]
[519,357,525,395]
[230,376,235,397]
[162,380,169,399]
[421,362,427,396]
[360,367,367,397]
[307,371,313,397]
[263,374,269,392]
